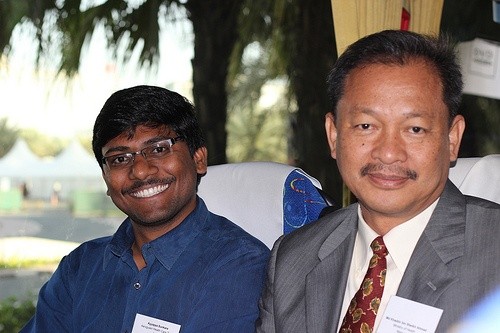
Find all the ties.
[336,235,389,333]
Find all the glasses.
[101,136,187,169]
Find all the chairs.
[449,154,499,204]
[196,160,333,251]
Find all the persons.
[255,30,499,333]
[15,86,271,333]
